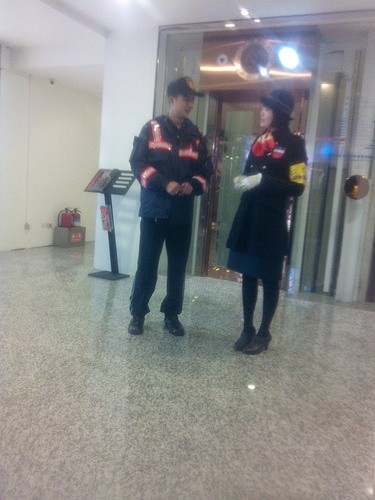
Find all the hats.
[167,77,204,97]
[261,89,295,121]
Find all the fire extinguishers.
[58,208,73,227]
[70,208,81,226]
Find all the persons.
[225,88,309,355]
[127,76,216,336]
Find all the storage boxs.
[54,226,86,247]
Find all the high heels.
[233,327,256,351]
[243,332,272,355]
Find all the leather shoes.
[164,314,185,336]
[128,317,143,335]
